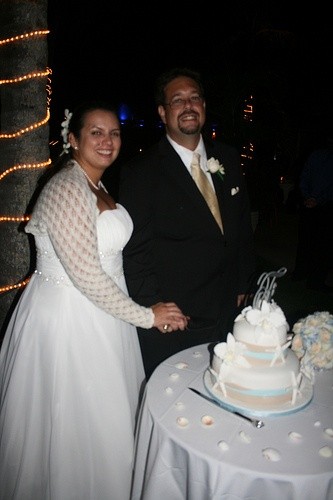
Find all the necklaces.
[66,159,102,191]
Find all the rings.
[163,323,169,330]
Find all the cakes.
[208,299,300,410]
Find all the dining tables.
[130,332,333,500]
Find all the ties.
[188,152,225,236]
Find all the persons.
[106,76,261,379]
[2,102,191,499]
